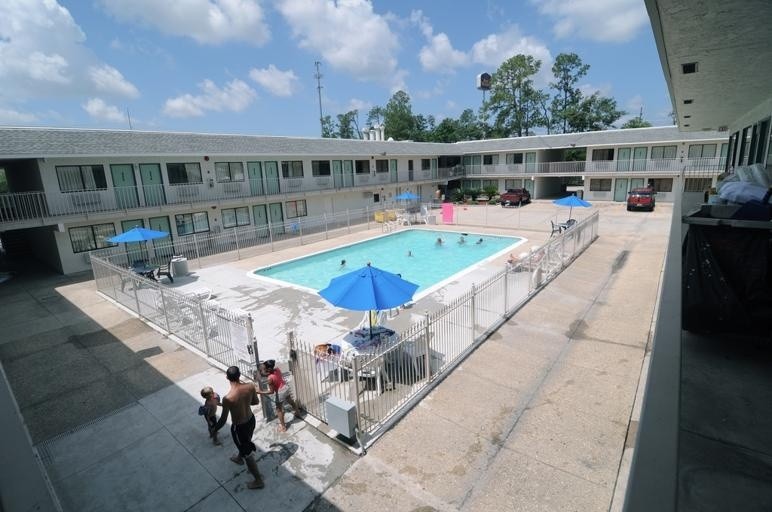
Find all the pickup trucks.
[500,188,531,207]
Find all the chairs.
[325,309,435,397]
[374,203,437,234]
[154,285,230,342]
[117,256,174,293]
[550,219,576,238]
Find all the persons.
[339,260,346,266]
[201,387,222,445]
[436,238,443,245]
[256,360,300,432]
[476,238,483,244]
[210,366,264,489]
[457,237,466,243]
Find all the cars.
[627,187,657,211]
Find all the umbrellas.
[317,262,420,340]
[395,193,419,212]
[106,225,170,268]
[552,193,592,222]
